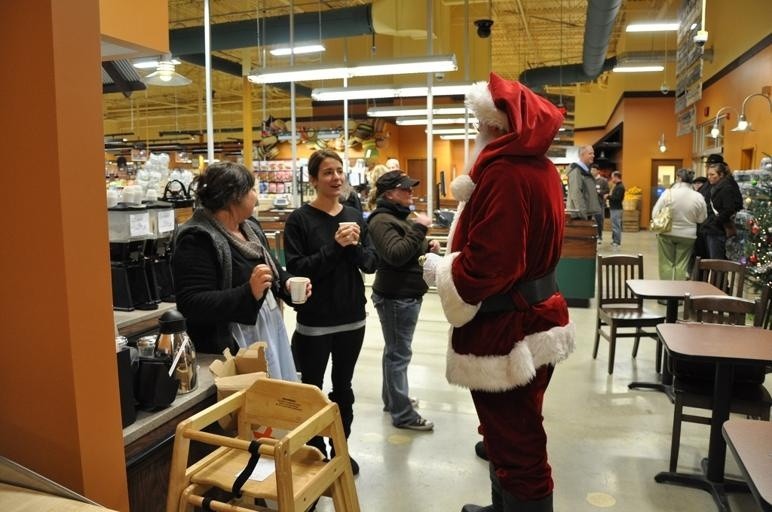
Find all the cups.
[288,276,310,305]
[107,185,159,209]
[338,221,358,245]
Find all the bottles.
[116,310,200,430]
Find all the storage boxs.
[208,341,270,431]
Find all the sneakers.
[386,397,433,430]
[658,299,683,305]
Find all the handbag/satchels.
[649,204,672,233]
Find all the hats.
[375,170,420,190]
[706,154,728,165]
[449,72,568,200]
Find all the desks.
[722,419,772,512]
[655,323,772,512]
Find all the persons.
[366,170,440,432]
[651,154,743,306]
[283,148,382,474]
[424,73,574,512]
[169,161,312,384]
[565,143,625,247]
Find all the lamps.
[626,21,679,33]
[658,134,667,153]
[132,56,183,68]
[247,0,478,141]
[705,106,751,139]
[614,63,664,74]
[730,94,772,131]
[139,50,193,87]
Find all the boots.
[461,462,553,512]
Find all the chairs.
[165,378,361,512]
[594,253,772,473]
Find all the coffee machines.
[105,200,181,312]
